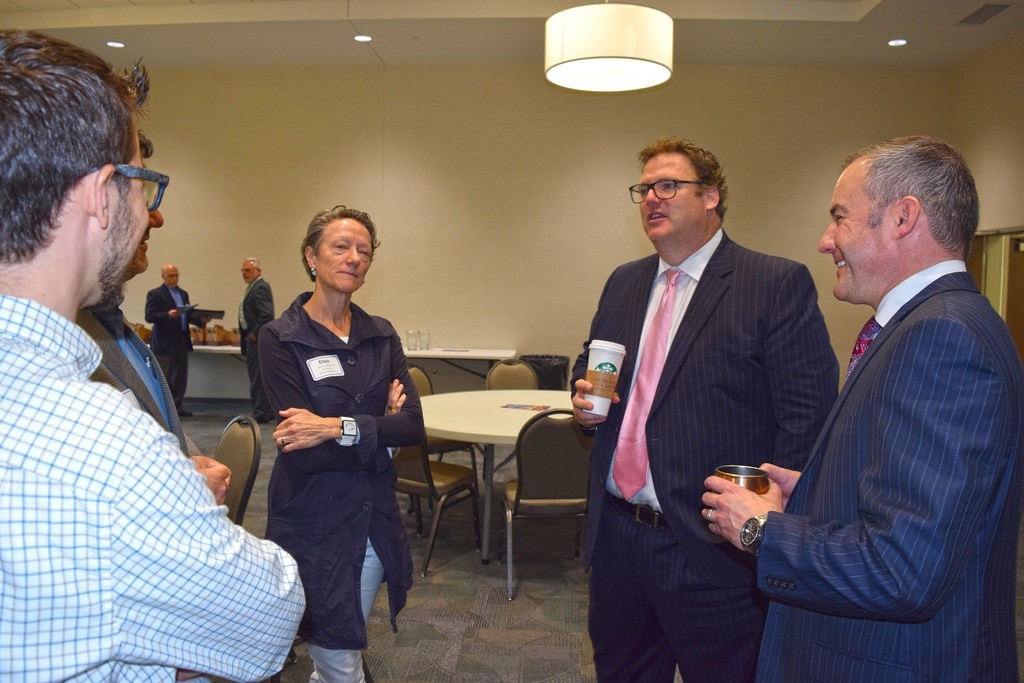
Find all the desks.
[419,389,574,565]
[193,346,247,364]
[403,347,517,379]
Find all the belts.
[602,493,672,529]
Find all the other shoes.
[242,418,267,423]
[179,411,192,415]
[269,414,274,420]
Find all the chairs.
[390,358,594,602]
[214,413,261,527]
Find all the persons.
[570,140,840,683]
[258,205,424,683]
[702,136,1024,683]
[145,264,211,417]
[0,29,305,683]
[238,258,275,425]
[74,131,232,506]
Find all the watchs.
[335,417,356,446]
[740,514,767,556]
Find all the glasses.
[629,180,707,204]
[164,273,180,277]
[90,164,170,211]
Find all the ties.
[614,271,681,501]
[847,316,884,384]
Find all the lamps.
[544,0,673,91]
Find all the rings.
[388,406,396,412]
[707,509,712,520]
[282,439,285,444]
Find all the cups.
[406,329,418,351]
[418,329,431,350]
[581,339,627,417]
[715,463,770,495]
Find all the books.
[191,309,224,319]
